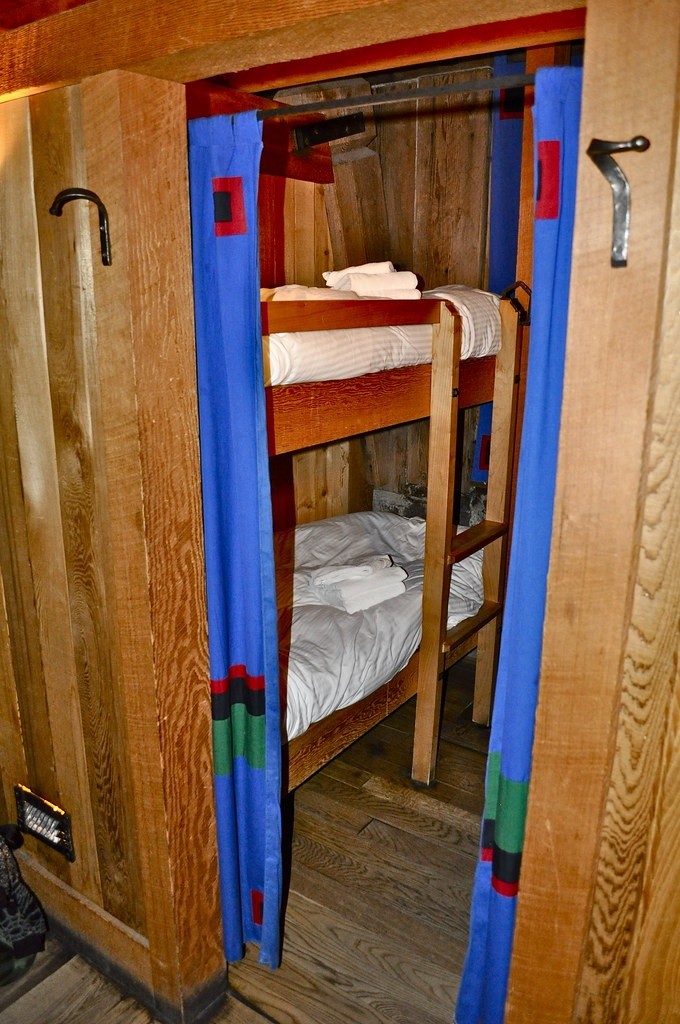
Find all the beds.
[261,284,526,795]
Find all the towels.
[308,553,409,615]
[321,260,423,301]
